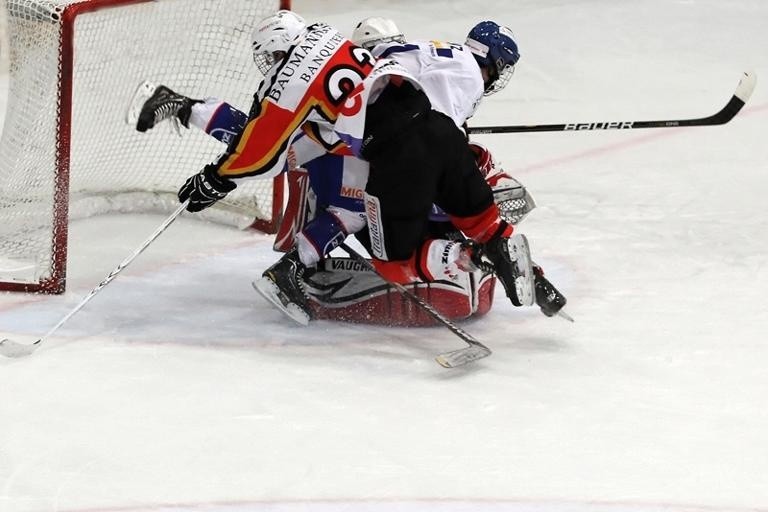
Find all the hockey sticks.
[338,242,491,368]
[466,71,756,133]
[0,198,189,358]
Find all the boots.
[135,84,204,137]
[260,243,315,322]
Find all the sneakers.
[469,235,568,318]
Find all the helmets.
[463,20,522,96]
[350,14,406,52]
[249,8,308,77]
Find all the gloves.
[176,162,237,213]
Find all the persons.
[125,10,575,325]
